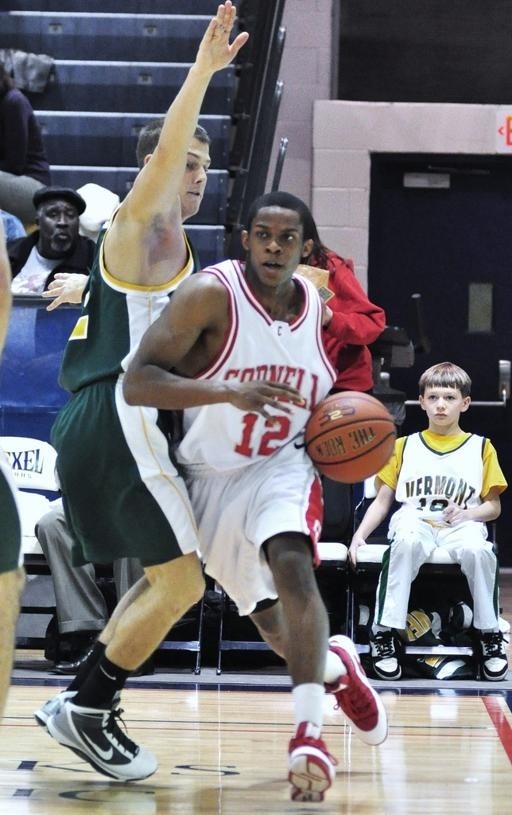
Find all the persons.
[0,0,388,804]
[346,364,509,681]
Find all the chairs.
[215,480,352,683]
[350,474,495,684]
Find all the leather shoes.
[50,646,94,676]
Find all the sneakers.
[286,721,340,804]
[324,634,388,747]
[478,632,510,683]
[34,690,80,727]
[369,622,404,682]
[46,697,160,784]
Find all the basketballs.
[303,390,396,483]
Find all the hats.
[33,187,87,216]
